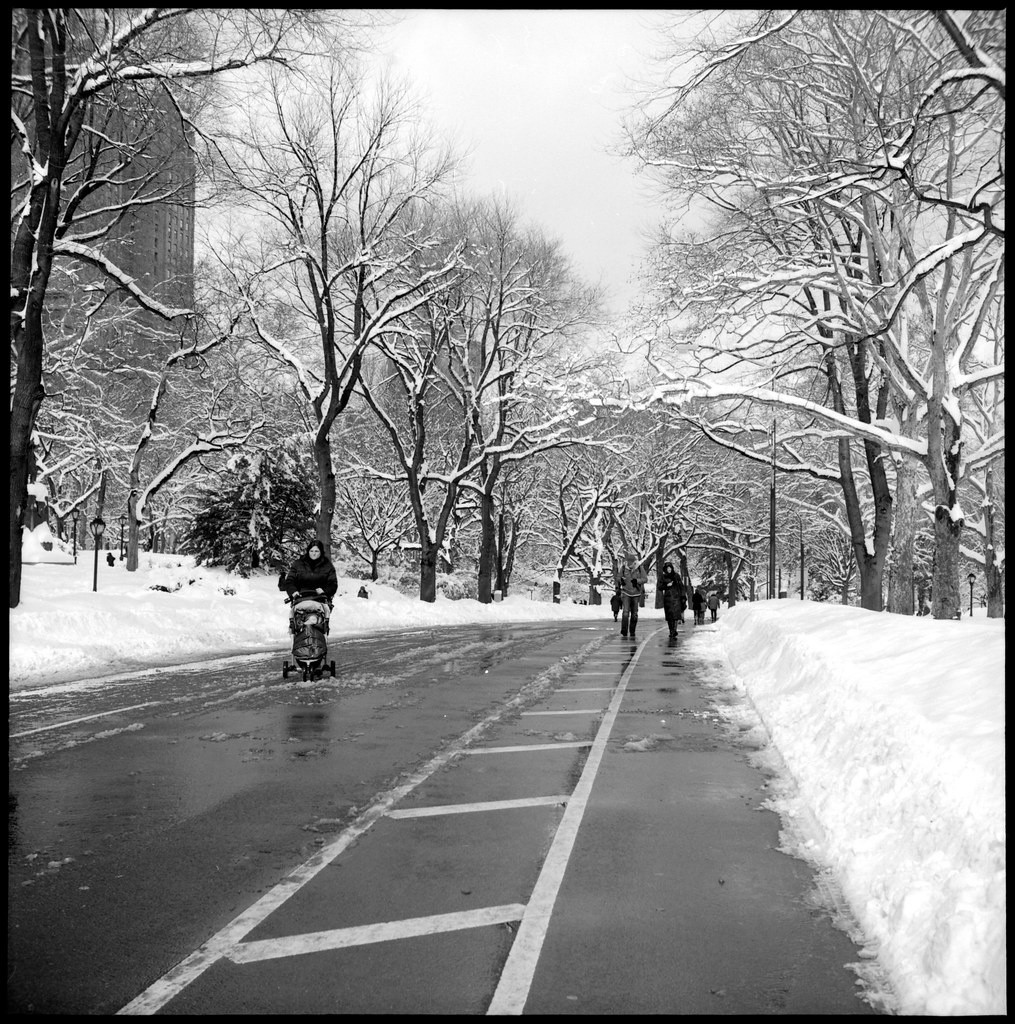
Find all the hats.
[625,555,637,565]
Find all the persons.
[106,552,116,567]
[692,585,721,625]
[277,540,338,659]
[742,594,747,601]
[619,554,648,641]
[573,599,588,605]
[610,590,623,621]
[357,586,369,599]
[657,562,687,638]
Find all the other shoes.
[630,633,635,636]
[621,630,628,636]
[669,629,678,638]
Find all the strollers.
[282,590,336,682]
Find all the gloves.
[631,579,637,585]
